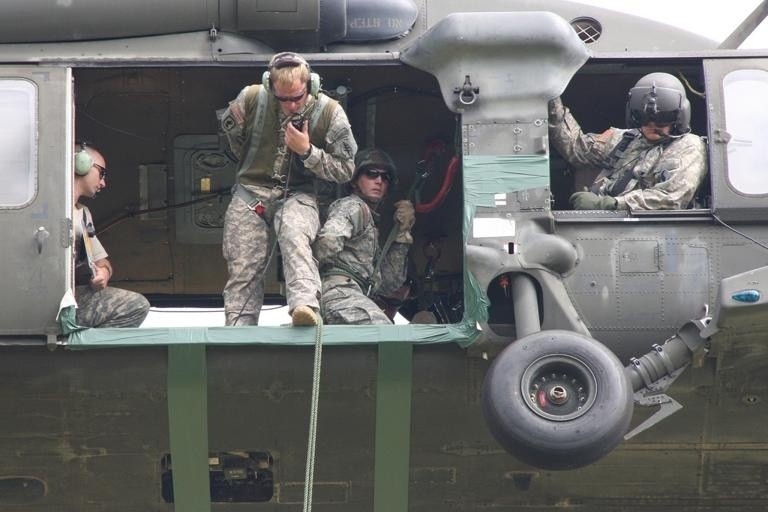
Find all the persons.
[216,51,356,326]
[74,138,150,328]
[548,72,708,211]
[312,146,415,324]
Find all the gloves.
[569,190,615,209]
[549,97,566,124]
[396,199,415,233]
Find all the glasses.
[634,109,677,127]
[273,92,305,103]
[366,168,390,182]
[93,162,107,179]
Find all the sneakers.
[292,305,318,326]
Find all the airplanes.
[1,2,768,512]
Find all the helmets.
[625,72,690,134]
[352,149,399,183]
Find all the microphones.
[300,96,318,117]
[654,127,691,138]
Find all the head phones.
[75,141,93,176]
[262,51,321,96]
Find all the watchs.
[300,149,311,161]
